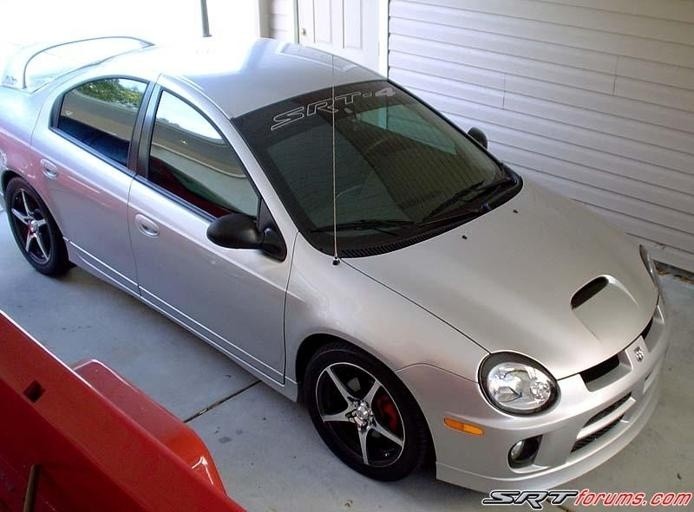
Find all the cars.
[0,33,672,499]
